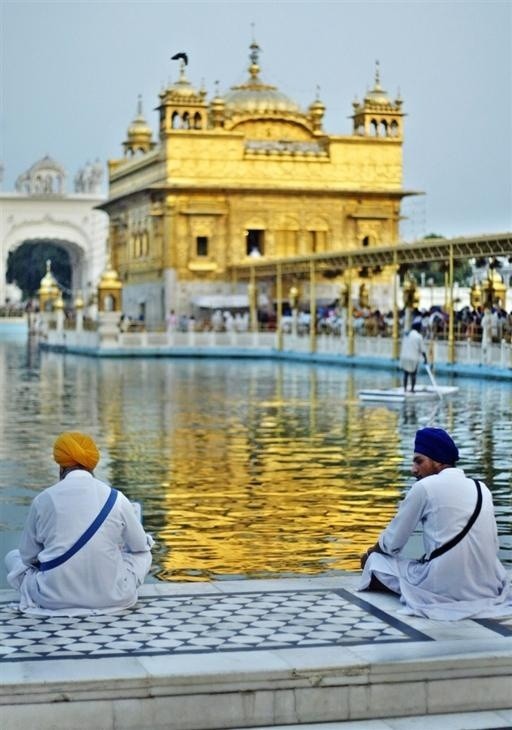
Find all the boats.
[356,385,459,402]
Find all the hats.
[53,431,100,470]
[414,428,460,465]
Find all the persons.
[317,301,349,337]
[399,323,428,394]
[166,303,277,333]
[356,427,512,623]
[353,301,512,345]
[281,302,310,336]
[5,431,156,616]
